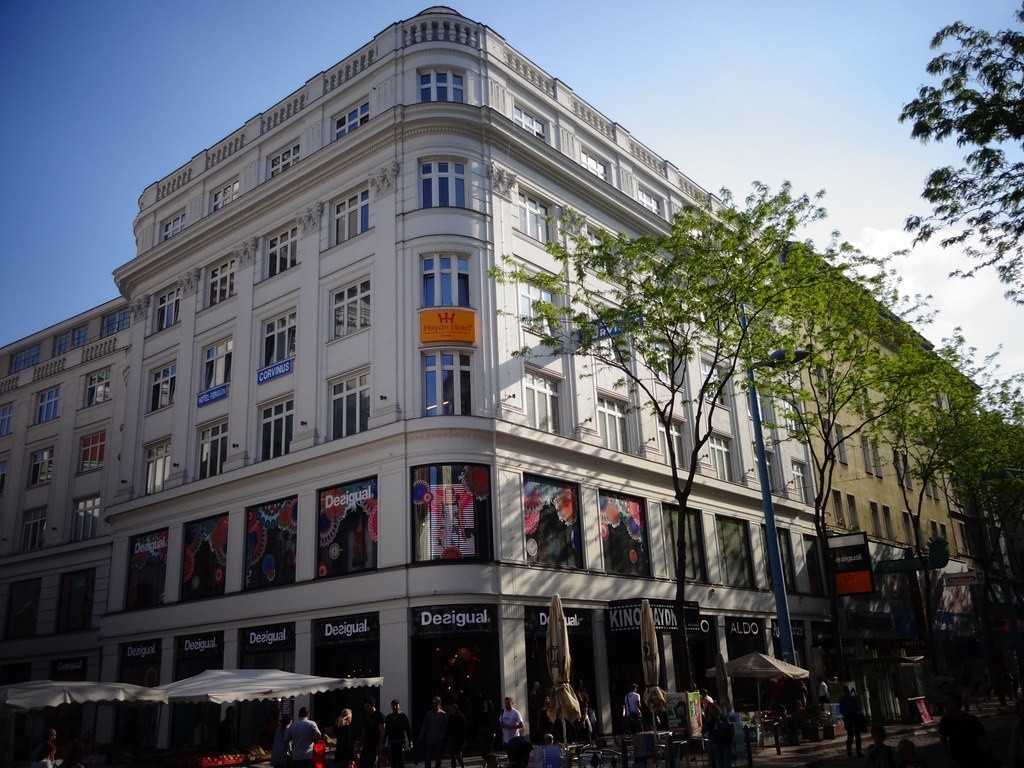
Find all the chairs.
[483,741,698,768]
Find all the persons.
[28,728,89,768]
[701,667,1024,768]
[261,696,563,768]
[573,691,595,746]
[625,684,644,735]
[191,706,237,754]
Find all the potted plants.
[799,711,825,742]
[816,711,836,740]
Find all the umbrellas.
[705,653,809,750]
[640,599,669,744]
[546,593,583,744]
[155,667,383,703]
[0,679,168,719]
[715,652,736,715]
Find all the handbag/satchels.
[628,711,643,724]
[860,713,868,733]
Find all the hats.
[546,733,554,739]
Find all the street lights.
[981,467,1024,692]
[747,349,809,706]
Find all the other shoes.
[857,752,864,758]
[847,750,852,757]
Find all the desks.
[207,758,271,768]
